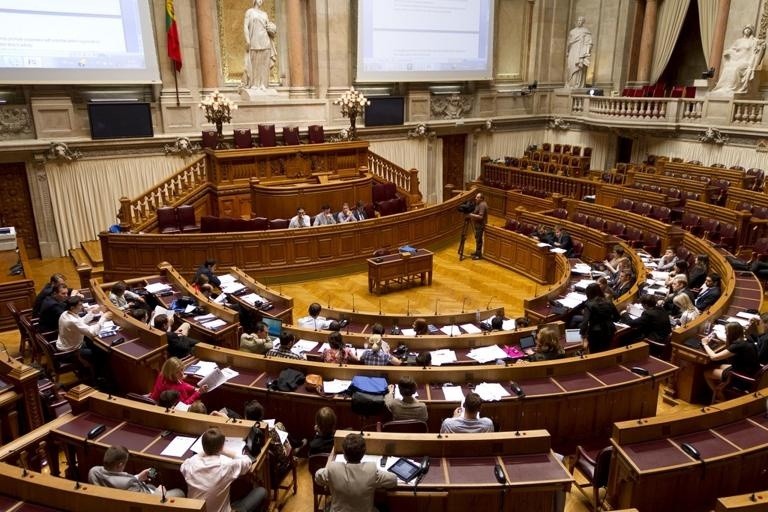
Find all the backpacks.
[272,368,304,391]
[242,422,264,455]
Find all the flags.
[164,0,183,73]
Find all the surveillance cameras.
[527,81,538,90]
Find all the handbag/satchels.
[305,375,334,397]
[347,375,388,414]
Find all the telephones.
[161,292,173,297]
[740,272,753,277]
[112,337,125,346]
[682,441,700,459]
[632,365,648,375]
[421,456,431,473]
[511,383,522,396]
[494,464,505,485]
[87,423,106,439]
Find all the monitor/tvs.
[364,94,405,127]
[87,102,154,139]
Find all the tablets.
[564,328,584,343]
[387,456,421,483]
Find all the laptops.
[261,316,282,340]
[519,335,535,354]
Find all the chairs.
[6,299,98,385]
[713,359,768,401]
[156,205,288,234]
[643,332,673,362]
[202,123,325,149]
[571,442,616,509]
[363,182,407,219]
[621,82,696,99]
[265,444,333,511]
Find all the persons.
[312,433,399,511]
[337,202,358,224]
[179,427,271,512]
[354,201,369,221]
[385,377,429,424]
[287,206,311,228]
[529,222,554,243]
[243,0,278,91]
[30,257,258,360]
[436,392,496,434]
[146,302,569,362]
[544,224,575,259]
[579,244,767,406]
[87,443,187,499]
[144,356,337,467]
[466,193,489,261]
[710,24,767,95]
[565,16,594,90]
[312,204,337,226]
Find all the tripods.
[457,215,476,261]
